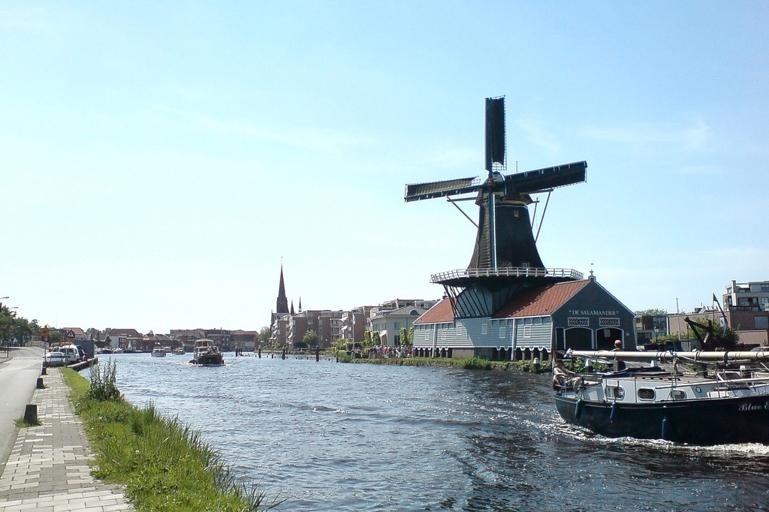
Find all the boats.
[551,341,769,444]
[152,339,224,367]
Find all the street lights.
[6,305,19,358]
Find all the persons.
[351,344,411,360]
[607,339,626,371]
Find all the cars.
[43,340,89,367]
